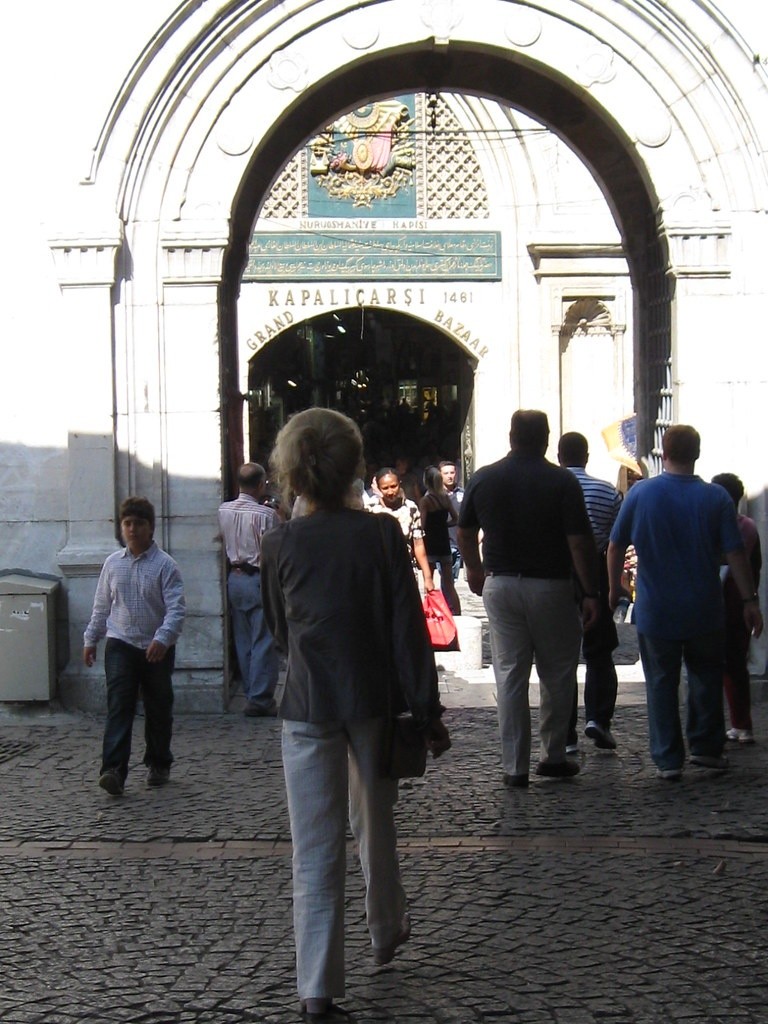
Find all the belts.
[487,570,566,580]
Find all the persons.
[257,409,454,1013]
[710,472,762,744]
[555,432,622,754]
[458,410,601,789]
[219,461,306,718]
[605,426,762,779]
[360,450,466,618]
[83,495,186,795]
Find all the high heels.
[375,913,410,965]
[300,997,331,1013]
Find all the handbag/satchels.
[377,713,429,780]
[423,589,457,645]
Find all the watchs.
[743,592,759,603]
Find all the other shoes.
[584,721,616,748]
[726,727,754,743]
[565,744,579,754]
[656,768,681,779]
[98,767,123,795]
[689,752,728,768]
[242,703,276,716]
[147,764,170,786]
[536,762,580,776]
[504,774,528,787]
